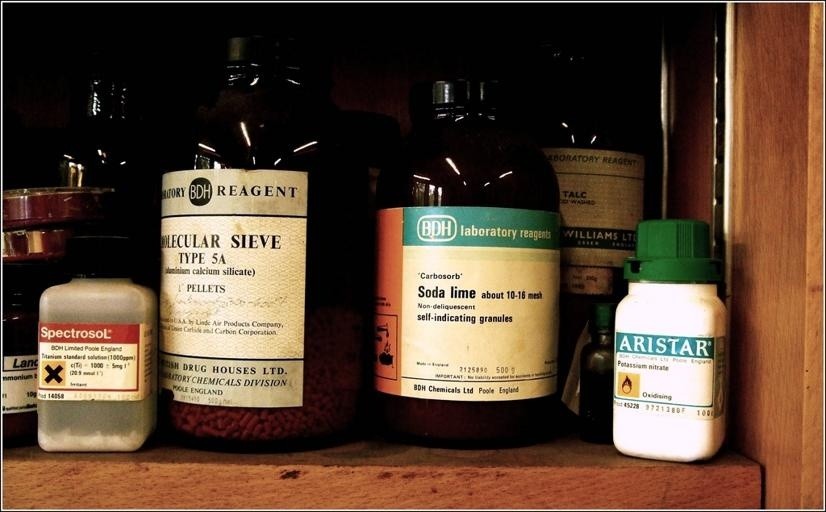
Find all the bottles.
[58,77,139,187]
[535,29,654,417]
[156,35,370,444]
[371,80,561,440]
[612,219,732,462]
[578,303,614,444]
[36,235,158,453]
[0,184,113,448]
